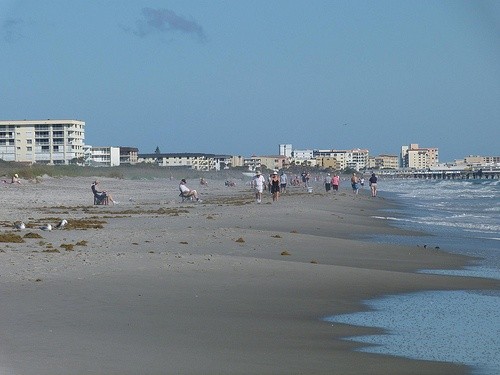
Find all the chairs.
[179,190,193,203]
[94,192,108,205]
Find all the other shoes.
[198,199,203,203]
[257,199,261,202]
[113,201,120,205]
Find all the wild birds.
[12,223,25,230]
[39,224,52,231]
[55,219,68,229]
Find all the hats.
[273,171,277,174]
[255,171,261,176]
[182,179,186,183]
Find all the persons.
[331,174,339,191]
[360,177,364,189]
[269,171,280,202]
[280,171,288,194]
[324,173,331,193]
[268,174,273,194]
[251,171,266,203]
[301,169,311,188]
[369,173,378,197]
[179,179,203,202]
[12,174,22,185]
[351,173,358,196]
[91,180,120,205]
